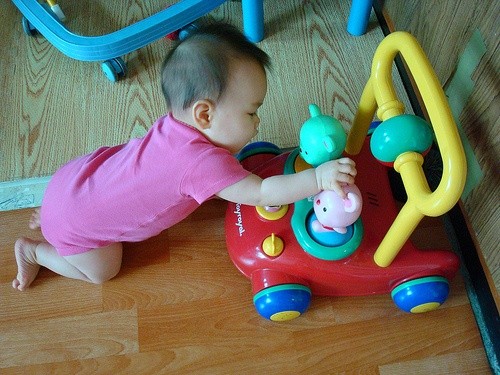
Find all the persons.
[12,25,357,291]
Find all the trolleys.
[225,31,468,323]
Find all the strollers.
[9,1,233,82]
